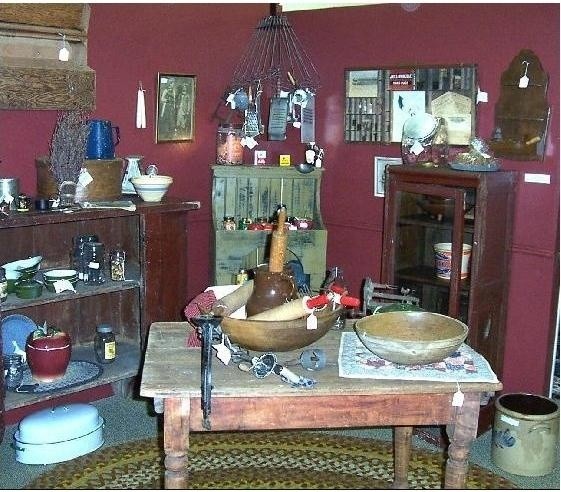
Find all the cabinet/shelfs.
[0,191,200,426]
[380,162,519,452]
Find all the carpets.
[25,433,523,489]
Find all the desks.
[138,319,505,489]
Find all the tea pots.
[14,194,31,213]
[86,119,120,160]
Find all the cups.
[36,199,49,210]
[3,354,23,391]
[457,138,495,165]
[73,233,105,286]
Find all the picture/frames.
[342,66,476,147]
[154,72,197,141]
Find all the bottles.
[110,250,126,281]
[216,123,243,165]
[400,113,447,168]
[305,142,318,165]
[220,216,250,230]
[324,265,346,330]
[93,325,117,363]
[0,268,8,301]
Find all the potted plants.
[24,320,71,382]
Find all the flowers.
[47,106,93,208]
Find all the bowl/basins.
[43,270,78,292]
[222,305,341,351]
[0,314,37,354]
[131,175,174,202]
[355,310,469,366]
